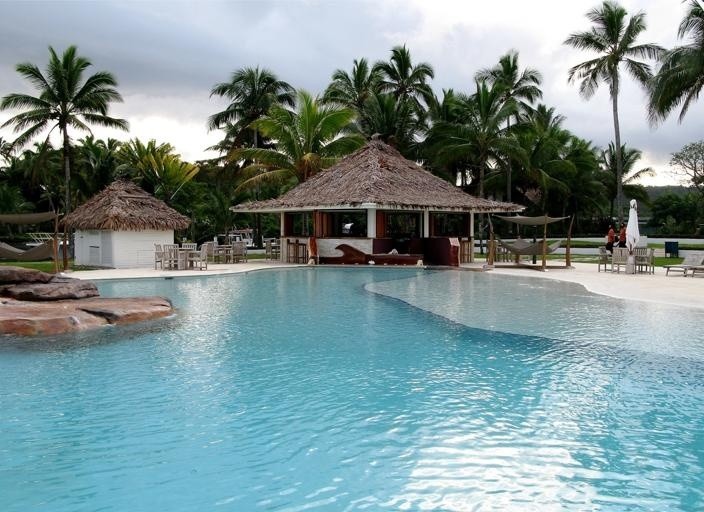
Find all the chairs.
[152,239,249,269]
[458,235,513,263]
[261,237,310,265]
[594,246,704,278]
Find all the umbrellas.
[624,199,639,256]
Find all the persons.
[618,222,627,248]
[605,224,615,255]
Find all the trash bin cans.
[665,241,678,258]
